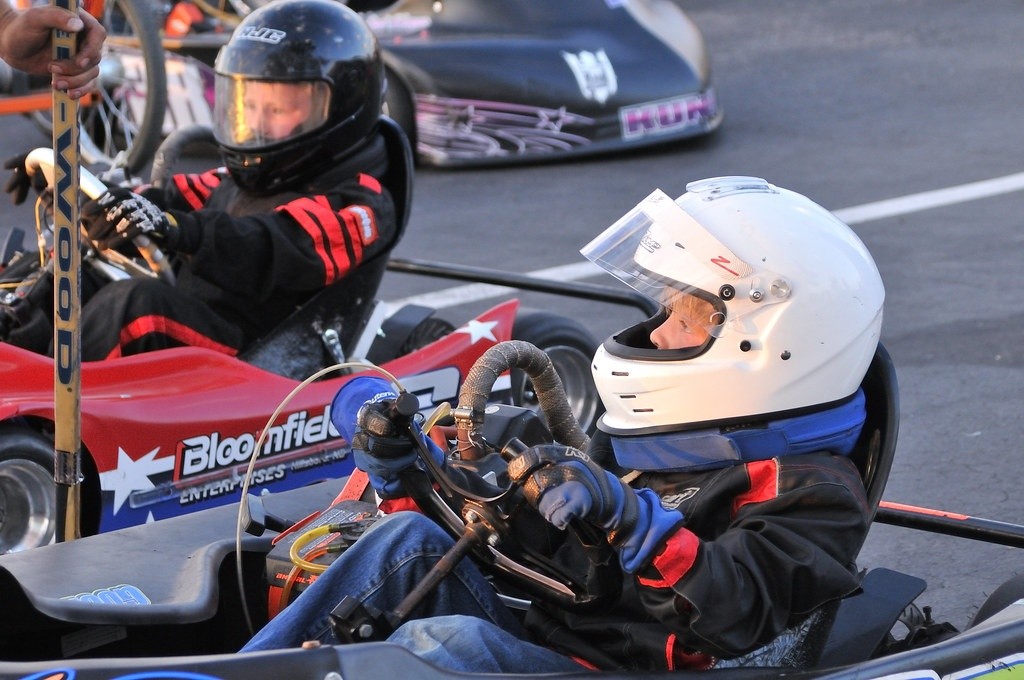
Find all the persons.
[0,1,399,363]
[244,283,870,675]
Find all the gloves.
[507,444,685,574]
[80,180,180,253]
[353,397,446,500]
[4,146,47,206]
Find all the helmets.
[214,0,387,198]
[591,176,886,470]
